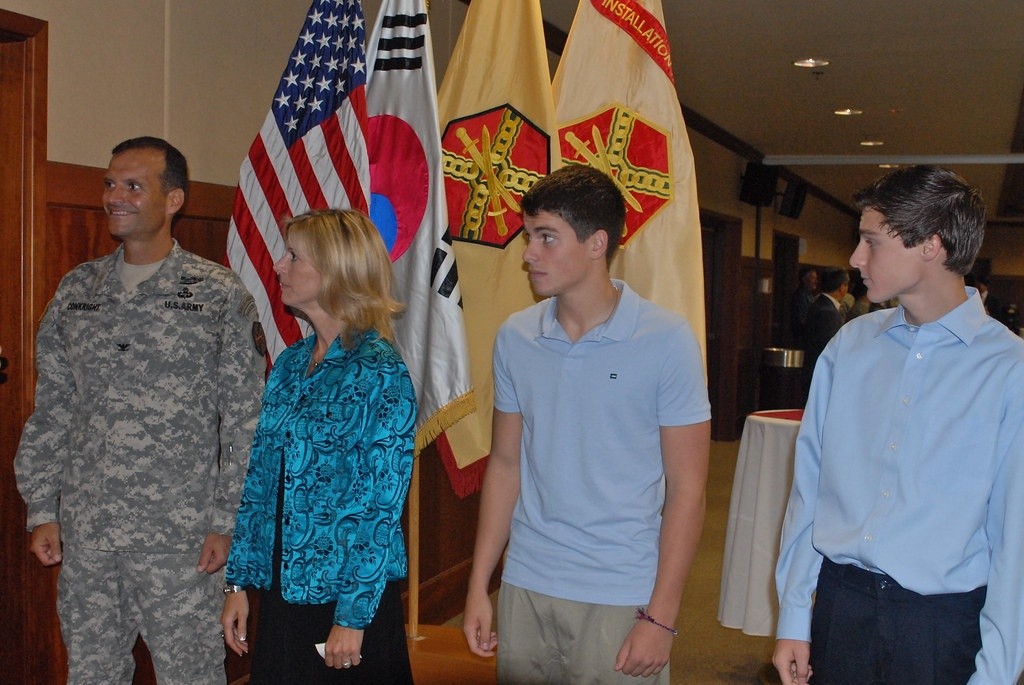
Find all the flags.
[432,1,560,501]
[553,0,706,417]
[366,0,475,462]
[227,0,369,374]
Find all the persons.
[771,166,1024,685]
[972,270,1024,340]
[15,136,267,685]
[221,209,416,685]
[789,268,817,342]
[801,266,850,407]
[464,164,711,685]
[840,292,899,326]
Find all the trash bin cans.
[762,347,805,410]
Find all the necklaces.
[605,285,619,324]
[312,358,319,367]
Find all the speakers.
[740,162,779,206]
[778,181,807,219]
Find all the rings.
[221,634,225,638]
[342,662,351,668]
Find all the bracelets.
[638,608,677,635]
[223,585,246,595]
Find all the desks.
[717,408,807,638]
